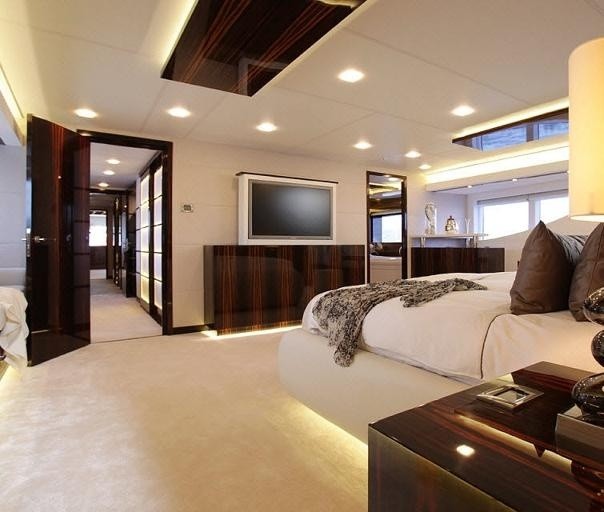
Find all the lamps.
[568,37,604,223]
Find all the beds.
[276,271,603,444]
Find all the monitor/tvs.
[247,179,334,241]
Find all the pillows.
[511,220,603,322]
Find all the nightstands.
[367,361,604,510]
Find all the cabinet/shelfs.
[203,244,365,336]
